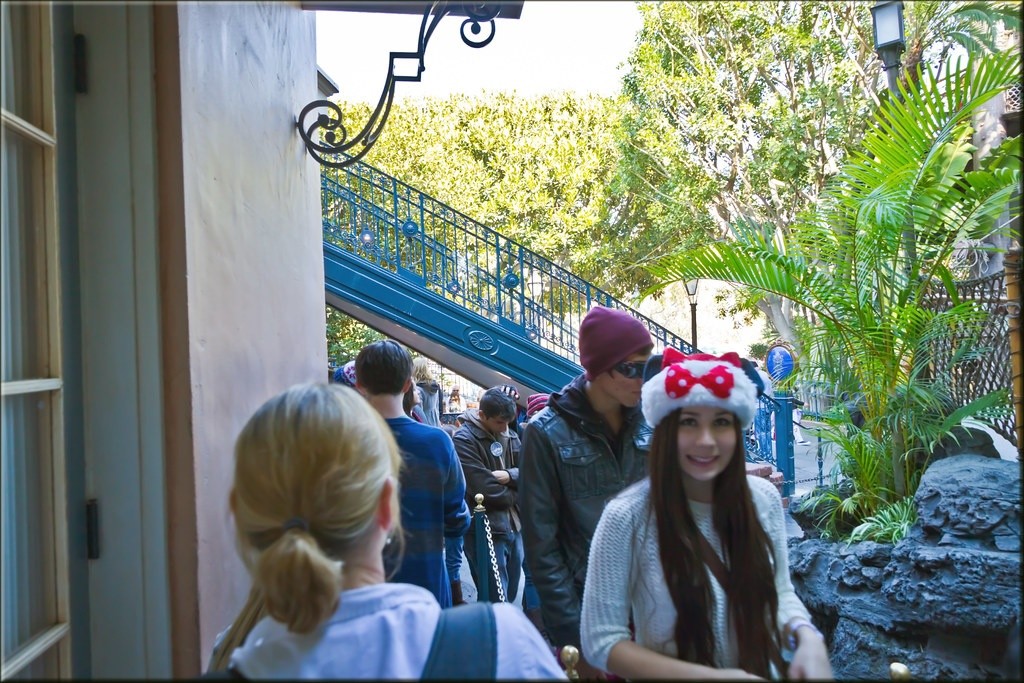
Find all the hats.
[577,305,651,379]
[641,347,766,430]
[498,385,520,399]
[526,392,551,416]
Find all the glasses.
[616,359,650,380]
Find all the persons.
[207,384,571,682]
[579,350,834,680]
[515,305,659,681]
[325,357,446,425]
[439,384,552,608]
[749,359,775,464]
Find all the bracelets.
[787,619,826,652]
